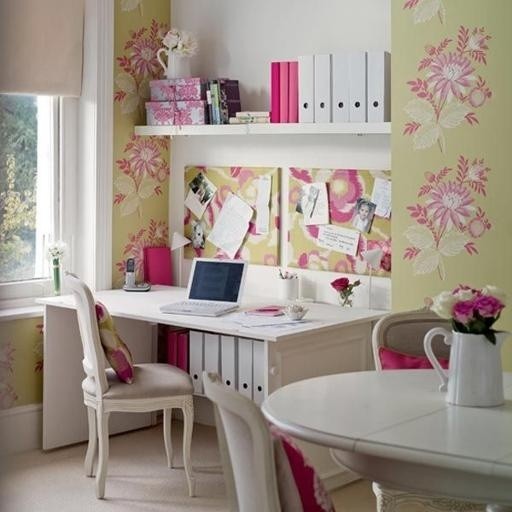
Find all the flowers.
[163,28,198,58]
[45,240,68,296]
[329,276,361,308]
[429,284,510,345]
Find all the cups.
[277,279,298,301]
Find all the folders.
[185,329,264,404]
[295,52,391,123]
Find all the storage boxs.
[149,77,206,101]
[144,100,208,126]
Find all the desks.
[260,368,512,512]
[34,283,391,494]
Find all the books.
[244,306,286,316]
[205,79,272,124]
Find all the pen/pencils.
[256,309,278,311]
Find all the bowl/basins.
[281,308,310,321]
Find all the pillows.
[379,347,449,369]
[94,300,133,384]
[270,424,336,512]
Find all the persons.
[352,200,372,232]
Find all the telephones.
[124,258,151,292]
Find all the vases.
[423,327,511,407]
[53,259,60,295]
[156,48,191,79]
[338,291,353,308]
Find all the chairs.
[371,306,452,512]
[202,371,281,512]
[62,271,196,499]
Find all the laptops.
[159,256,248,318]
